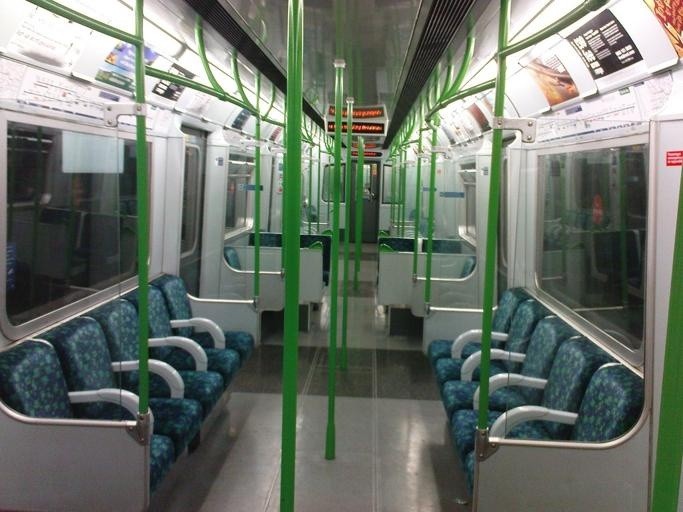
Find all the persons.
[420,222,435,237]
[302,202,308,222]
[409,209,424,221]
[363,183,370,196]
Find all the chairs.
[124,282,243,389]
[460,257,476,277]
[0,335,178,486]
[451,331,608,468]
[430,292,528,366]
[88,295,227,417]
[460,360,646,502]
[441,312,576,419]
[49,313,206,452]
[151,275,256,367]
[224,246,242,271]
[435,299,550,396]
